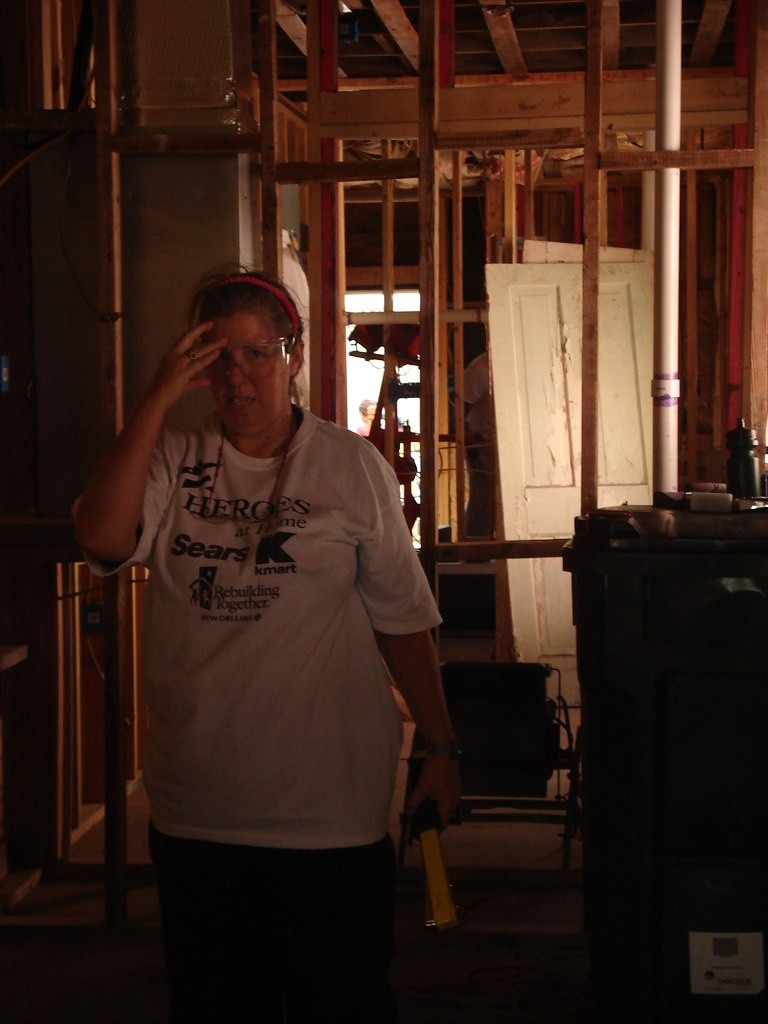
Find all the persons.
[71,264,459,1024]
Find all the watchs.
[427,741,460,759]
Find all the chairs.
[400,661,580,866]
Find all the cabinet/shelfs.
[561,537,767,1024]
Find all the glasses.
[203,449,287,524]
[202,337,295,385]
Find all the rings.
[186,347,196,361]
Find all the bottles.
[724,417,762,498]
